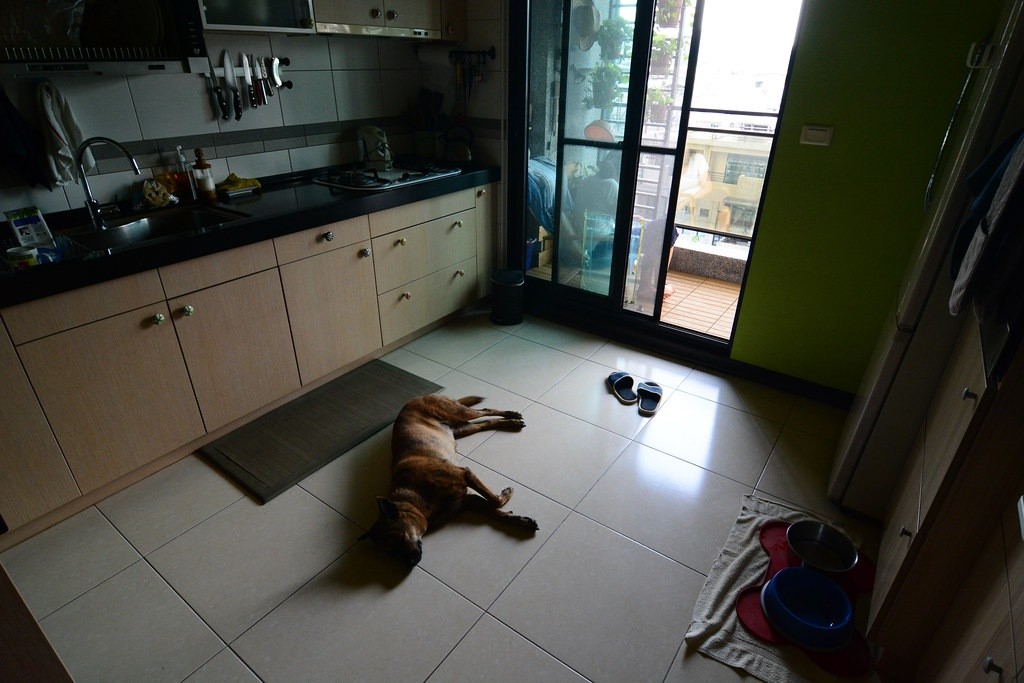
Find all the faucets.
[75,136,142,221]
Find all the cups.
[6,245,53,271]
[151,165,178,193]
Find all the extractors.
[0,0,211,80]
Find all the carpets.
[194,358,446,506]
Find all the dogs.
[358,395,540,570]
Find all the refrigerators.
[825,0,1024,519]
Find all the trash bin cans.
[490,268,526,326]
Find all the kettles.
[438,124,475,170]
[356,124,394,171]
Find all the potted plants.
[652,0,692,29]
[649,30,689,78]
[582,61,629,112]
[595,15,636,65]
[645,87,675,124]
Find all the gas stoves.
[311,164,461,190]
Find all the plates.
[217,186,257,196]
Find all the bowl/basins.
[787,521,859,572]
[764,567,853,646]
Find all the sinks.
[89,204,246,261]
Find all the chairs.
[579,207,646,308]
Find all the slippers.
[608,371,637,404]
[637,381,662,414]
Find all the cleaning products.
[149,142,218,202]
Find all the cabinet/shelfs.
[272,214,384,392]
[368,188,481,356]
[0,238,303,552]
[861,303,1024,683]
[313,0,442,44]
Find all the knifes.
[203,50,273,120]
